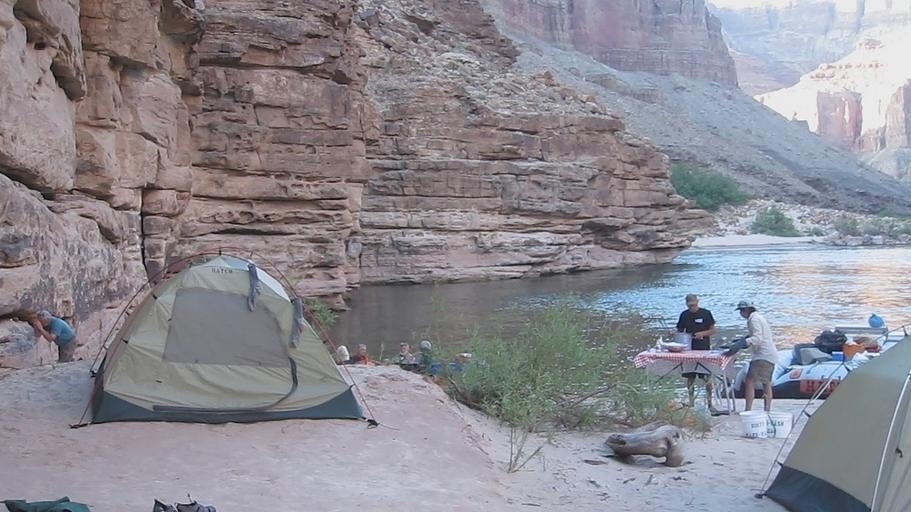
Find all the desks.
[645,349,737,415]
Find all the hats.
[735,301,760,311]
[337,345,350,362]
[687,294,698,305]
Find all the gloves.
[719,336,752,357]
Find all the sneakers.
[177,501,216,512]
[154,500,176,512]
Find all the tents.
[62,244,382,429]
[761,319,911,510]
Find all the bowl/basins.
[662,341,689,352]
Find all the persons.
[334,344,353,365]
[393,342,417,364]
[676,292,721,413]
[721,298,781,412]
[417,340,434,363]
[351,343,369,364]
[30,310,78,362]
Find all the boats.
[730,341,894,401]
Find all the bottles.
[655,341,661,353]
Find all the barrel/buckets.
[673,331,692,351]
[767,410,793,439]
[738,409,767,439]
